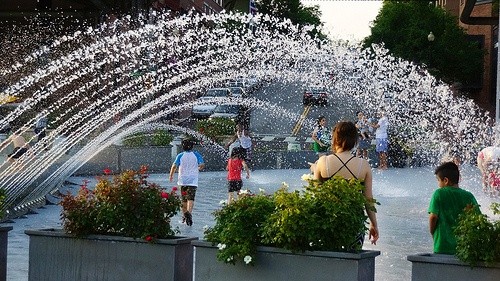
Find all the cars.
[192,76,264,129]
[301,70,337,105]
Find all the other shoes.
[183,211,192,226]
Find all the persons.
[313,121,380,250]
[426,162,481,256]
[226,147,251,201]
[477,147,500,198]
[224,124,257,173]
[312,110,389,171]
[33,112,49,156]
[169,139,204,226]
[6,127,32,165]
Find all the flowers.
[201,173,382,268]
[56,165,191,245]
[185,118,217,146]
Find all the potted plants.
[118,127,181,174]
[191,239,381,281]
[192,116,237,145]
[406,201,500,281]
[24,227,199,281]
[0,186,13,281]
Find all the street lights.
[427,31,435,67]
